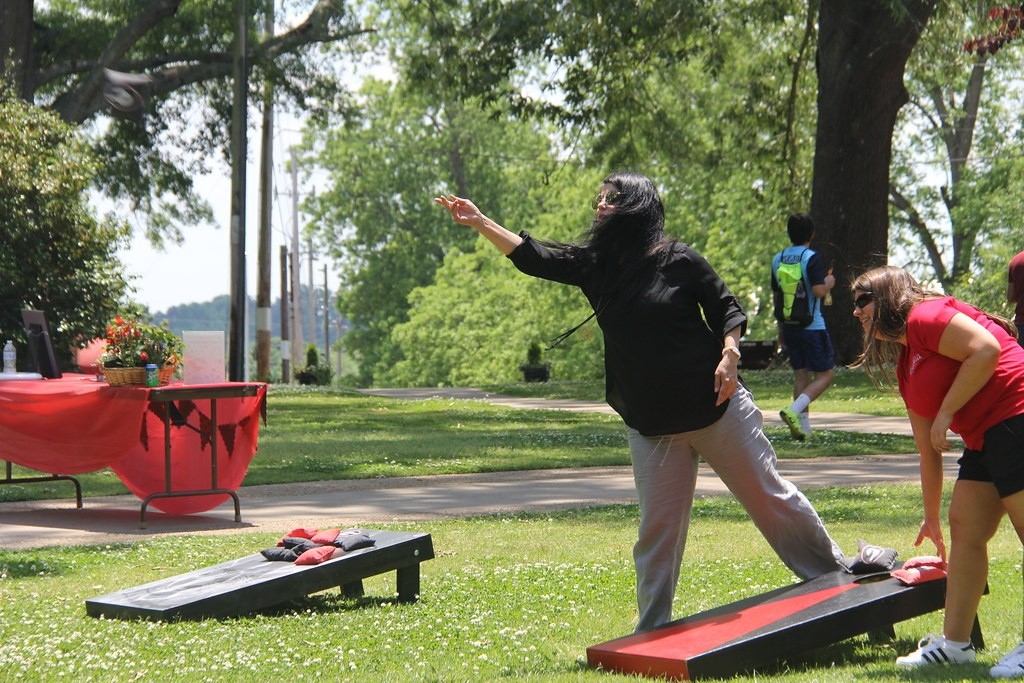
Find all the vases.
[102,365,175,387]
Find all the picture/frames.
[21,310,62,378]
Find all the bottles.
[3,340,16,373]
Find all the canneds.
[145,364,159,387]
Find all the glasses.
[592,190,622,210]
[854,292,876,308]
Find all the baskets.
[94,356,177,386]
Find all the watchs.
[722,346,741,360]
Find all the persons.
[851,265,1024,679]
[1007,250,1024,349]
[433,171,848,634]
[770,212,835,440]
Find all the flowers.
[91,316,184,368]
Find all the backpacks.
[774,247,817,331]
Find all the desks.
[0,371,269,530]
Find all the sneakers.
[896,633,976,667]
[989,642,1024,679]
[804,428,835,442]
[779,405,805,442]
